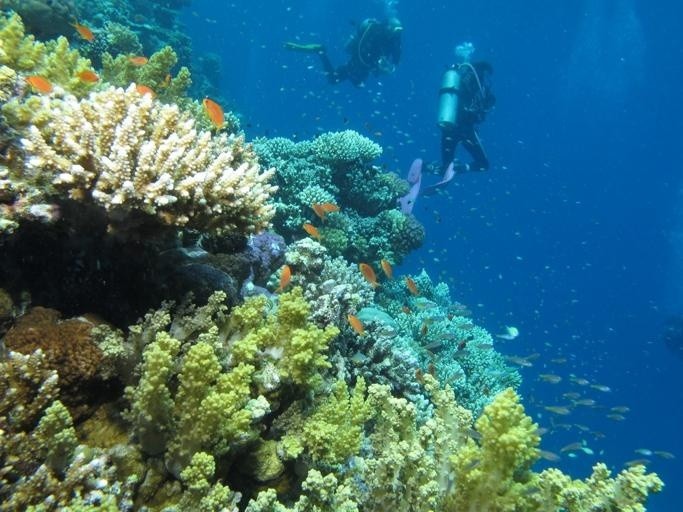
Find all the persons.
[307,18,403,87]
[407,64,496,184]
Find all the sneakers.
[454,162,462,173]
[420,161,427,173]
[314,45,325,53]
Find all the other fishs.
[415,308,676,512]
